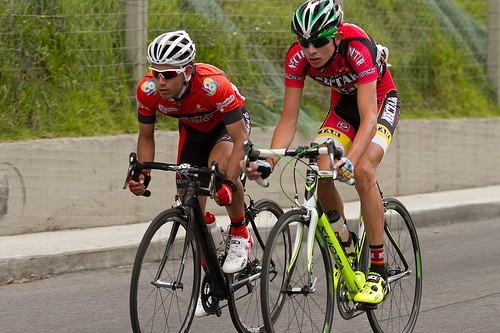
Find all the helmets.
[146,30,196,66]
[290,0,344,40]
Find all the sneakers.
[194,288,212,317]
[332,232,359,290]
[222,232,253,274]
[353,271,389,304]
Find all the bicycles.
[242,138,423,333]
[123,152,292,333]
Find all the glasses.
[149,64,190,79]
[299,32,337,48]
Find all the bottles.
[204,212,225,253]
[328,210,354,255]
